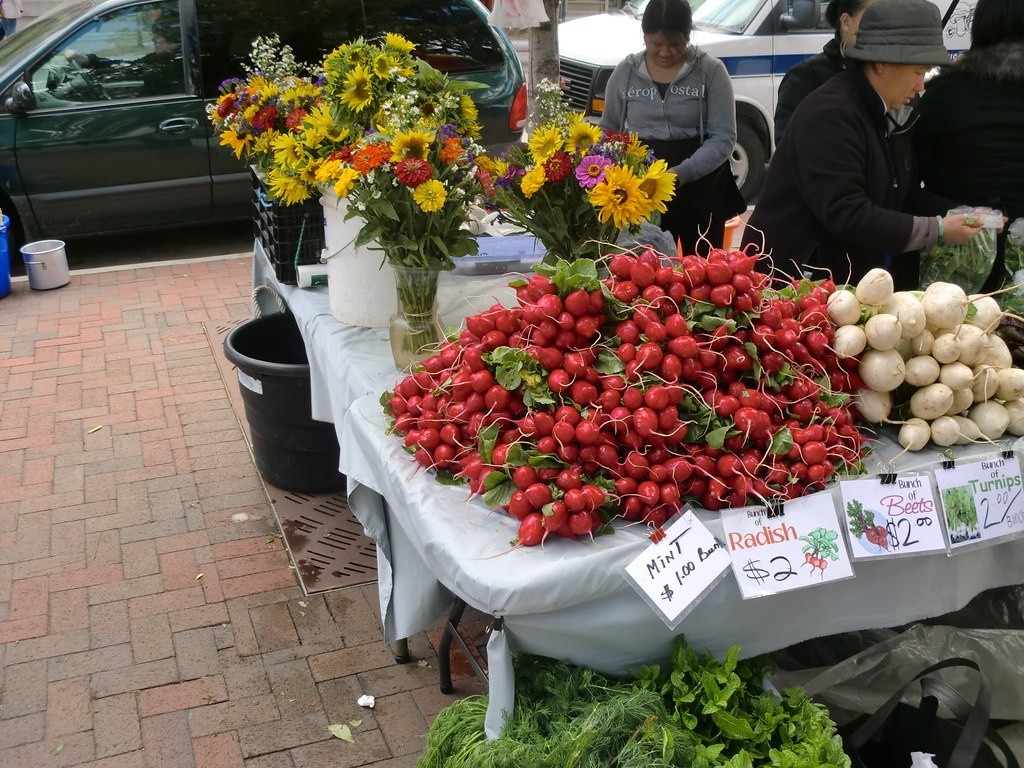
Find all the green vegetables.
[917,217,1024,315]
[414,634,853,768]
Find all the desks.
[250,237,1024,745]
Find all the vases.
[387,257,446,374]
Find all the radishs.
[828,267,1024,476]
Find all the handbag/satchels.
[835,657,1020,768]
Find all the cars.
[1,0,528,274]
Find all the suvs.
[558,0,978,203]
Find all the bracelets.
[935,215,943,245]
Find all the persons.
[740,0,1024,291]
[65,9,186,98]
[596,0,747,259]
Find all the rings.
[966,217,976,225]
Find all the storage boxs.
[248,163,326,283]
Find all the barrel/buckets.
[317,175,469,330]
[222,308,345,495]
[19,239,72,291]
[0,214,12,300]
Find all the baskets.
[247,162,325,284]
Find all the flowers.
[205,37,678,251]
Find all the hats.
[844,0,954,66]
